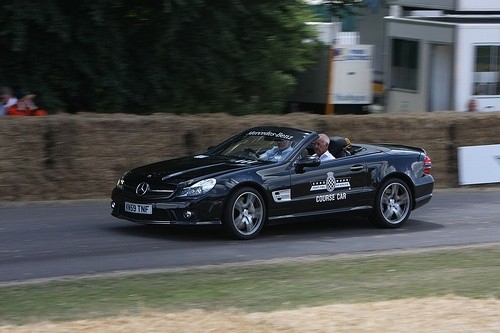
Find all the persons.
[309,133,334,162]
[464,99,479,111]
[249,138,294,161]
[0,86,47,115]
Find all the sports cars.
[110,126,434,241]
